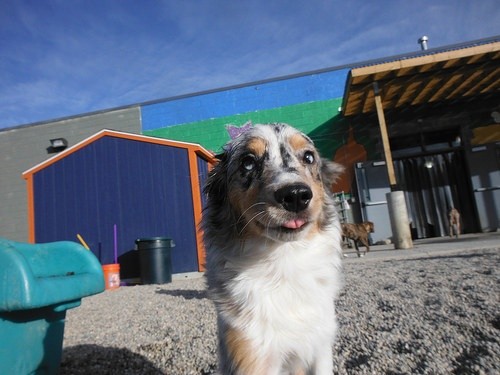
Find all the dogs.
[196,123,346,375]
[339,221,374,257]
[447,208,461,240]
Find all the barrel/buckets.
[102,264,121,290]
[136,237,172,284]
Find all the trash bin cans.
[136,236,175,284]
